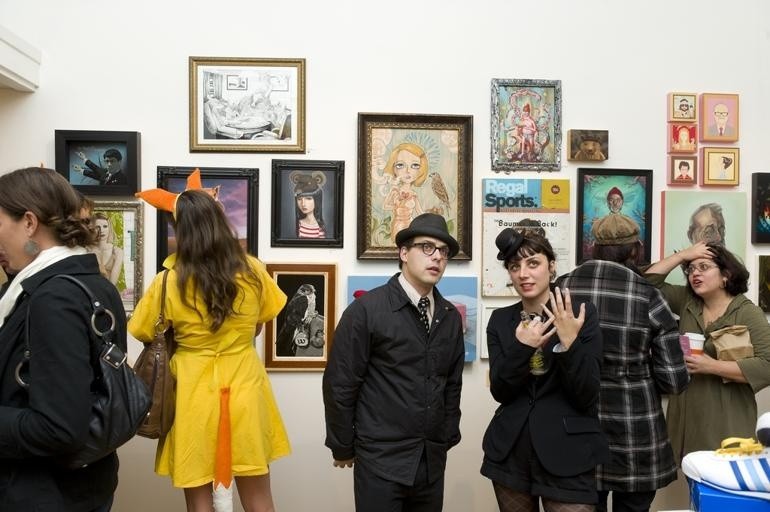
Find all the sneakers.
[681,438,770,499]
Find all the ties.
[417,298,431,336]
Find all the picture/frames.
[489,78,562,172]
[346,274,478,364]
[481,305,518,359]
[269,158,344,249]
[356,110,473,261]
[188,56,307,154]
[155,165,259,279]
[566,129,610,161]
[54,129,141,198]
[265,263,339,371]
[575,168,654,271]
[87,201,144,321]
[481,177,571,297]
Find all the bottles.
[520,310,531,327]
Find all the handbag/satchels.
[133,333,175,440]
[60,342,154,472]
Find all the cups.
[682,332,706,355]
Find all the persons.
[479,227,606,511]
[127,169,291,512]
[675,99,693,116]
[716,156,732,179]
[553,214,690,511]
[636,241,769,468]
[710,104,734,136]
[0,190,96,299]
[673,126,695,151]
[607,186,624,216]
[87,214,124,285]
[293,178,328,238]
[320,214,465,511]
[1,167,154,511]
[676,160,693,181]
[72,149,126,183]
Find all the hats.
[591,212,642,246]
[495,228,527,261]
[607,187,623,200]
[395,213,459,259]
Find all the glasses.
[400,239,450,257]
[715,112,728,117]
[683,262,719,276]
